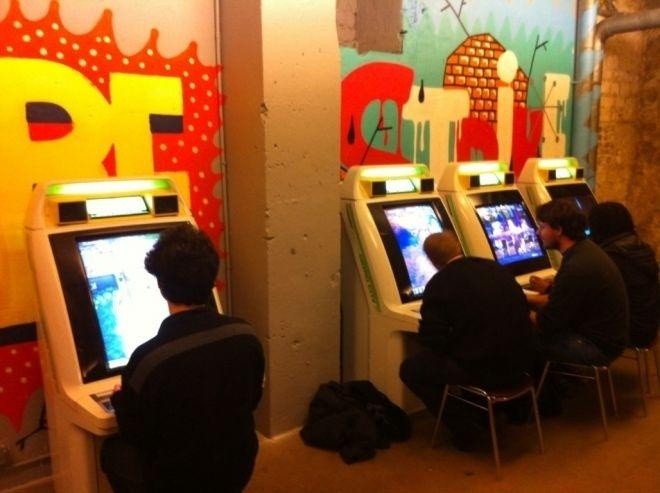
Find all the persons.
[520,198,630,415]
[100,223,265,492]
[590,200,660,348]
[399,233,533,451]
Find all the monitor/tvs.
[384,205,444,295]
[476,203,544,266]
[554,195,592,236]
[78,233,171,370]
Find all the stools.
[430,342,660,478]
